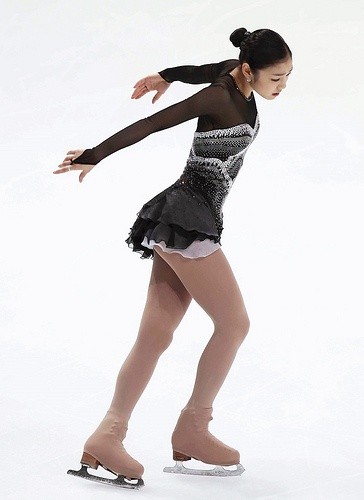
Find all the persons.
[52,28,293,491]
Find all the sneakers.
[163,408,246,476]
[66,418,145,490]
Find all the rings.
[68,166,70,171]
[145,86,147,89]
